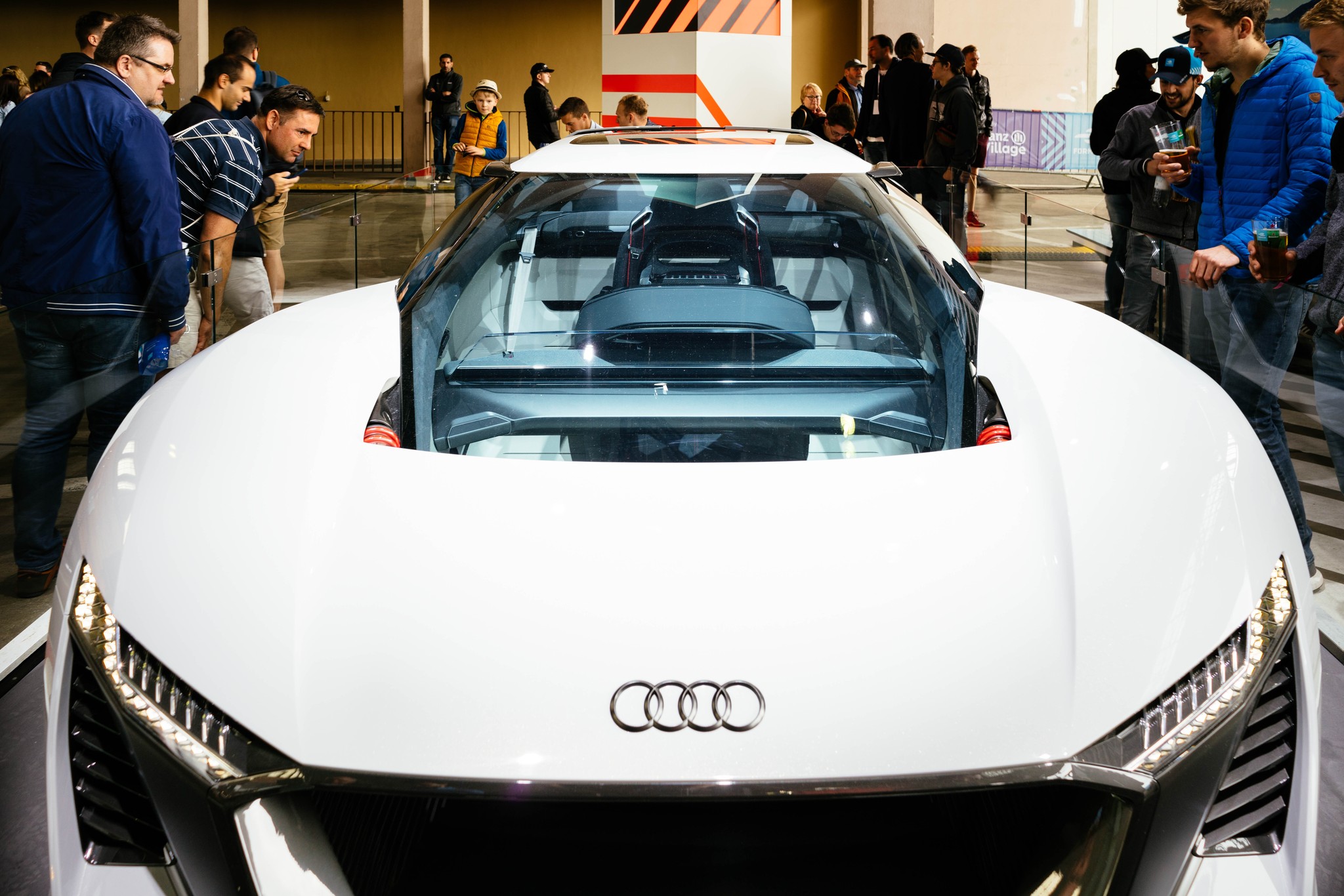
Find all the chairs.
[612,175,775,290]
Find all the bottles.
[1153,175,1172,209]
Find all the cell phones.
[287,168,308,179]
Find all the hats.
[470,79,502,101]
[1115,48,1158,75]
[1173,30,1191,44]
[530,62,555,75]
[845,58,867,69]
[925,43,966,70]
[1148,45,1202,86]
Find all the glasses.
[36,61,45,66]
[830,126,849,137]
[274,90,313,102]
[805,95,823,101]
[252,47,260,52]
[129,54,173,75]
[932,61,941,67]
[2,67,14,71]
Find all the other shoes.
[1309,562,1324,592]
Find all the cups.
[1149,120,1193,178]
[1252,216,1290,280]
[1172,191,1189,202]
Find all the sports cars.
[43,125,1321,896]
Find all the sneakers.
[0,533,68,598]
[965,213,987,227]
[432,173,451,183]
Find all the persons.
[523,63,562,150]
[164,83,323,380]
[0,16,189,595]
[1090,49,1159,321]
[854,34,900,178]
[450,79,508,209]
[791,83,827,136]
[0,61,51,124]
[878,32,936,217]
[959,44,993,226]
[426,53,463,183]
[825,59,867,155]
[222,25,292,312]
[917,43,978,255]
[1247,0,1344,505]
[1152,0,1344,591]
[50,7,119,84]
[616,94,675,132]
[162,50,301,336]
[1097,45,1205,372]
[812,103,863,160]
[557,97,647,199]
